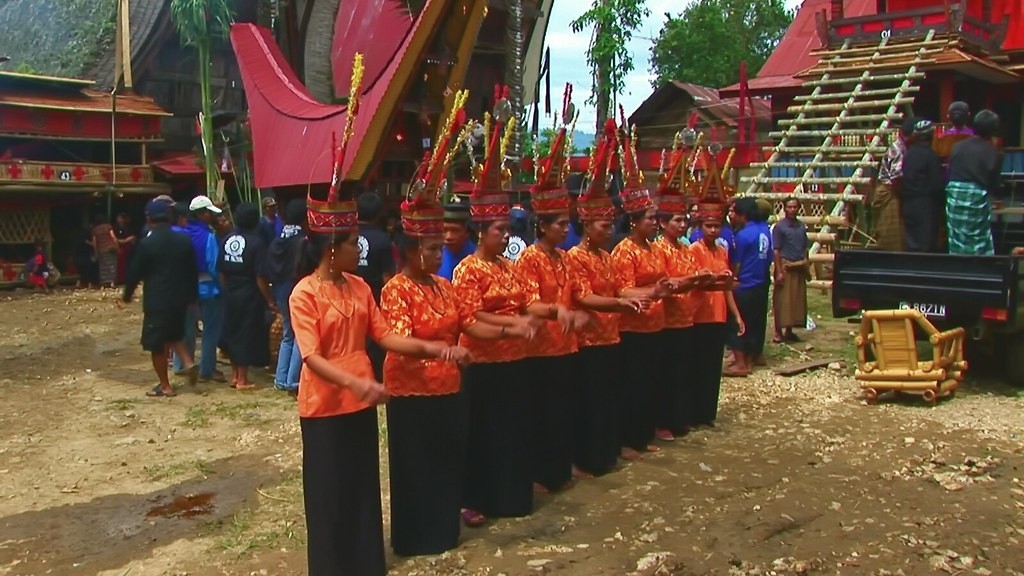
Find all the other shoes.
[272,383,301,392]
[204,369,229,384]
[656,427,676,441]
[720,365,748,377]
[461,506,488,529]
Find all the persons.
[772,197,813,343]
[137,194,477,389]
[20,196,137,293]
[452,187,775,527]
[2,148,26,160]
[117,199,199,397]
[289,197,450,576]
[877,100,1005,255]
[382,195,535,557]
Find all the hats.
[470,84,515,223]
[530,83,575,215]
[190,195,223,213]
[402,89,469,237]
[305,53,364,232]
[619,104,654,212]
[657,111,702,216]
[262,196,277,206]
[701,140,735,222]
[440,152,470,221]
[576,118,620,221]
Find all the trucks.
[831,145,1024,389]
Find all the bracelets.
[501,324,507,339]
[695,269,699,276]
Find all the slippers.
[174,363,198,377]
[144,383,177,398]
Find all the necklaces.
[403,271,446,316]
[579,241,610,280]
[314,268,355,319]
[698,237,717,258]
[478,249,513,291]
[538,242,566,287]
[627,236,656,269]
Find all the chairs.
[854,308,968,407]
